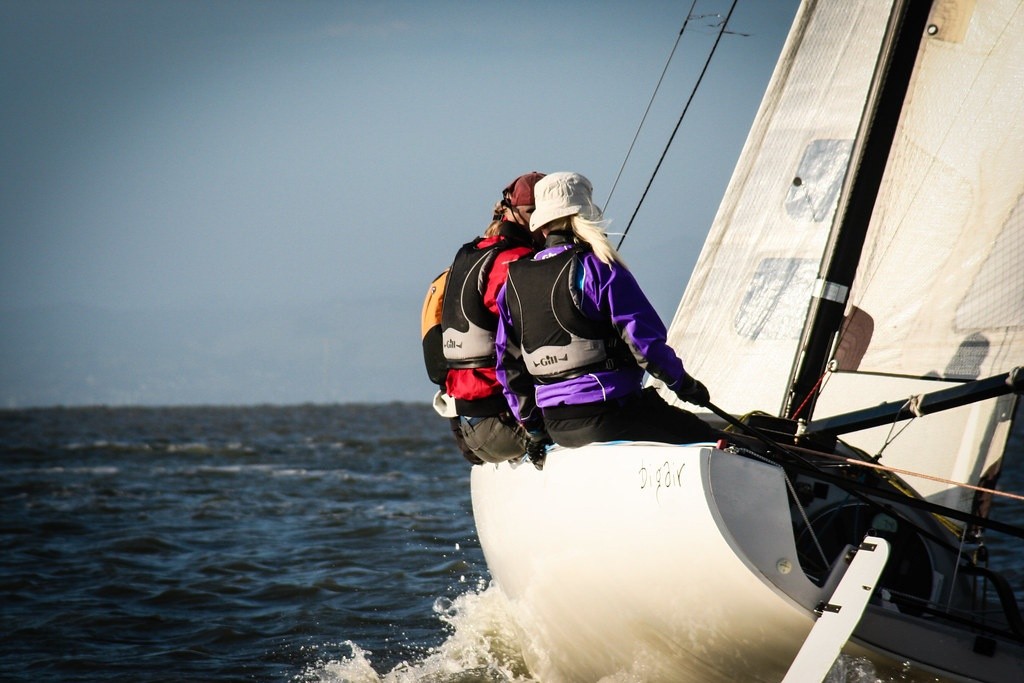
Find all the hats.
[530,172,600,232]
[502,172,547,207]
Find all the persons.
[493,169,720,472]
[421,170,555,466]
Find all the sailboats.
[467,2,1024,683]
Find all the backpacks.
[420,268,450,386]
